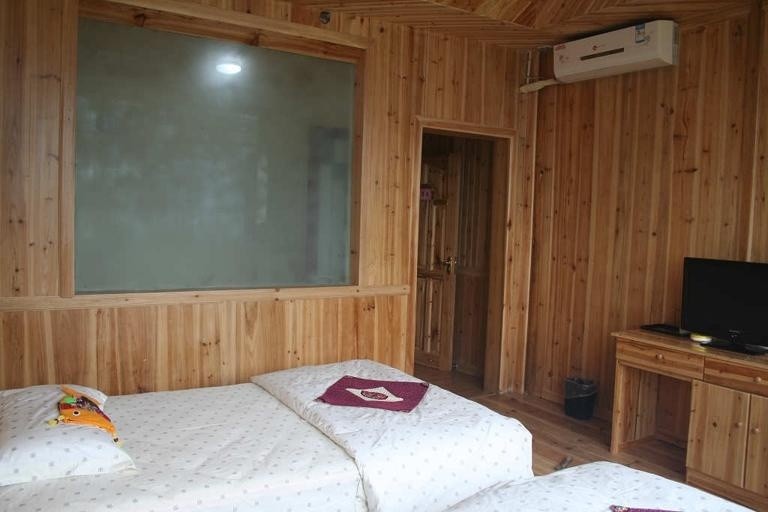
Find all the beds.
[0,361,534,512]
[446,460,768,512]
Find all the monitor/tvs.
[680,257,768,355]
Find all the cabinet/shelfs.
[612,326,768,512]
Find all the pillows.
[0,383,135,484]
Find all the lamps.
[217,60,241,75]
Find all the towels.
[318,376,429,413]
[610,504,671,512]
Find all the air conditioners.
[553,19,681,85]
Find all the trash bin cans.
[566,377,598,420]
